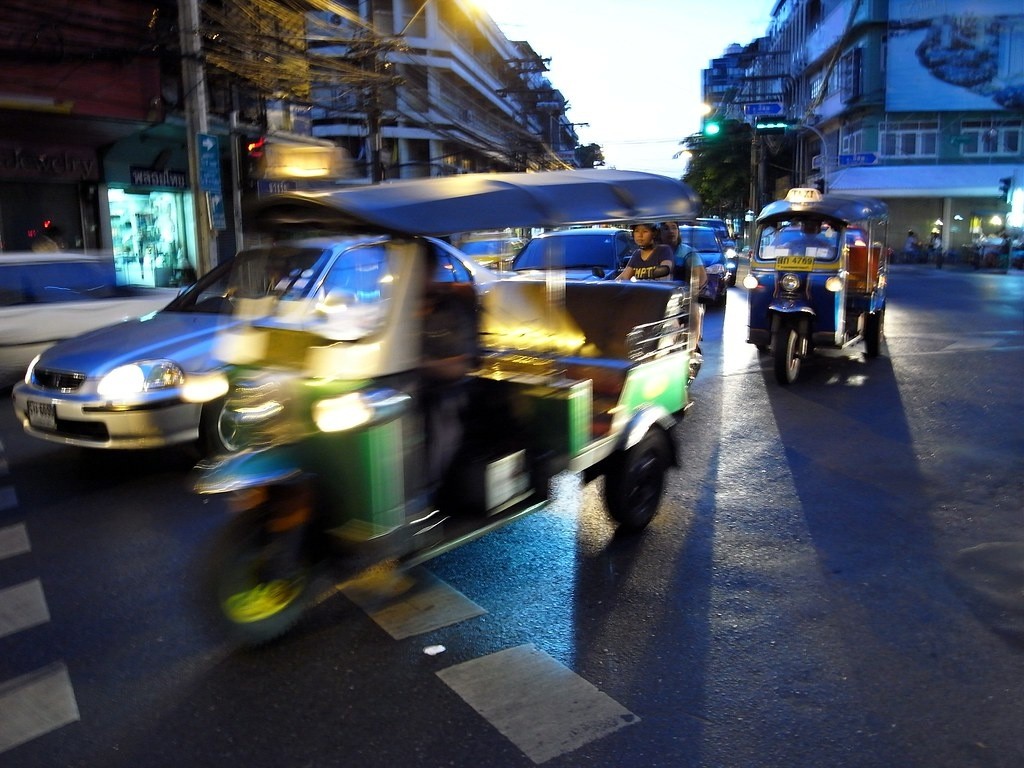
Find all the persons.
[904,232,921,262]
[615,223,673,281]
[403,240,481,506]
[781,220,830,255]
[924,235,940,263]
[658,222,707,351]
[984,233,1009,268]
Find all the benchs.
[845,246,884,293]
[466,276,686,403]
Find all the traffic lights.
[997,176,1017,207]
[755,117,790,138]
[235,136,264,189]
[704,121,742,138]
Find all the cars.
[0,253,192,387]
[451,233,528,270]
[511,227,641,285]
[14,234,497,451]
[674,217,740,306]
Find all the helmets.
[630,223,658,233]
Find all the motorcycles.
[745,187,894,384]
[206,169,706,632]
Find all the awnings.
[829,164,1024,198]
